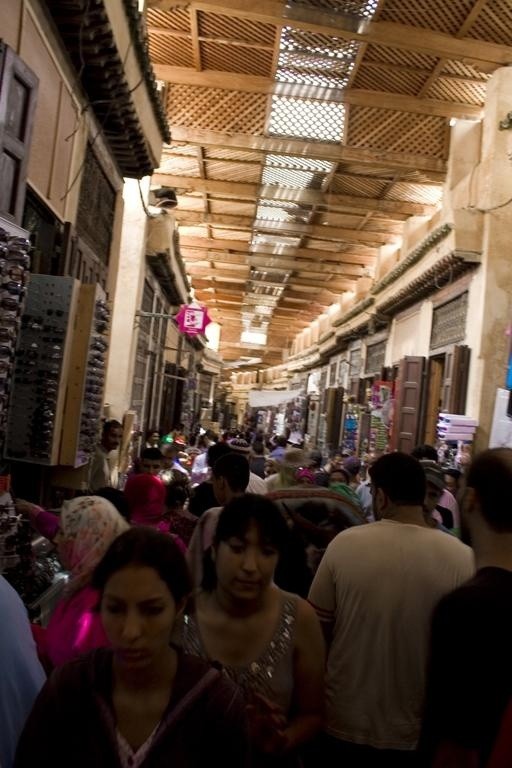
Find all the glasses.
[0,224,66,465]
[78,298,111,453]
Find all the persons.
[0,572,47,768]
[304,452,475,768]
[410,446,512,768]
[10,527,283,768]
[0,420,463,591]
[178,491,329,768]
[30,495,132,670]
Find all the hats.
[420,460,447,491]
[229,437,255,456]
[277,447,317,470]
